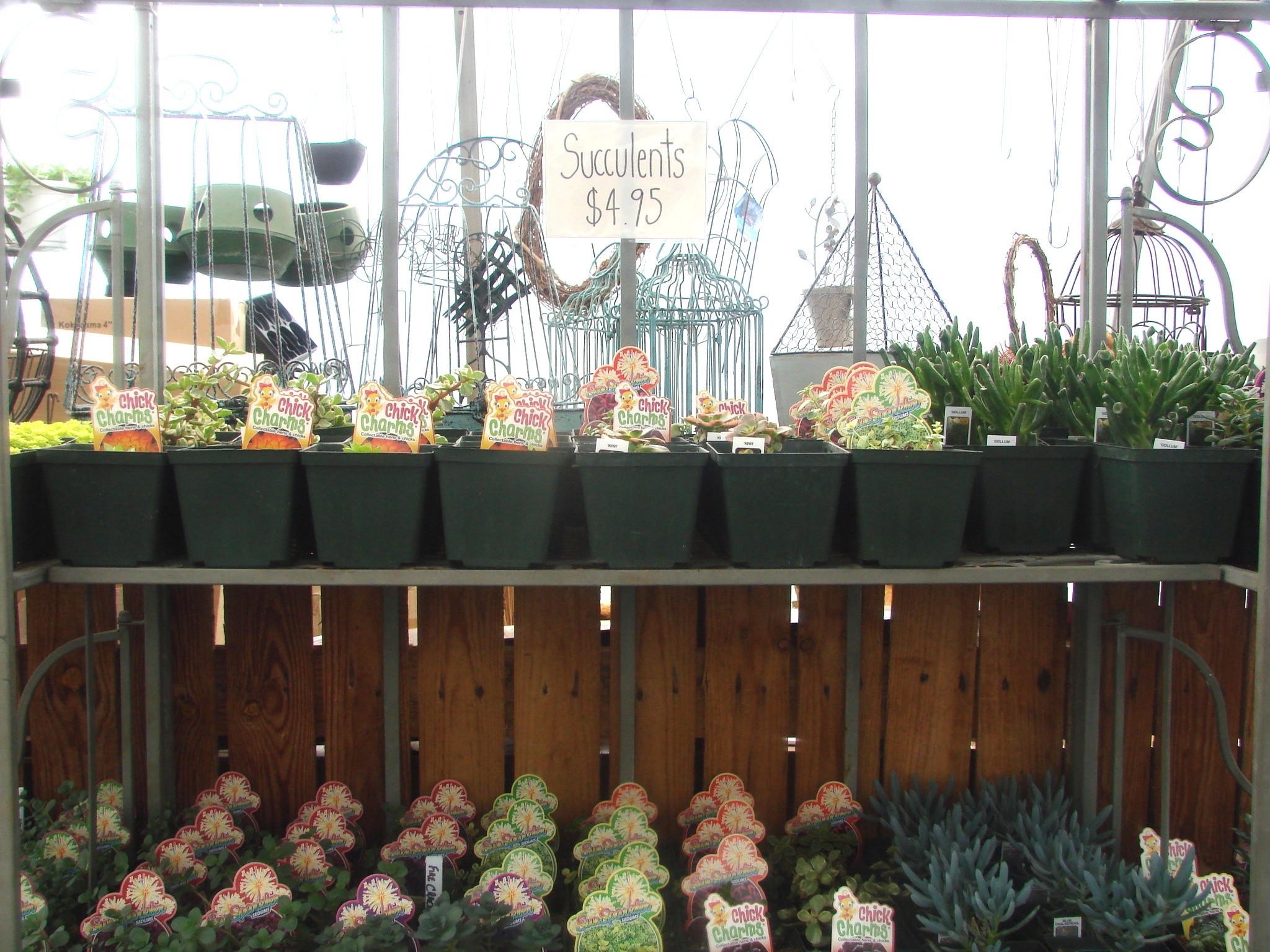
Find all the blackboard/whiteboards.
[542,119,707,239]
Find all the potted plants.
[20,771,1249,952]
[9,316,1266,569]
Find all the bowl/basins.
[301,139,367,185]
